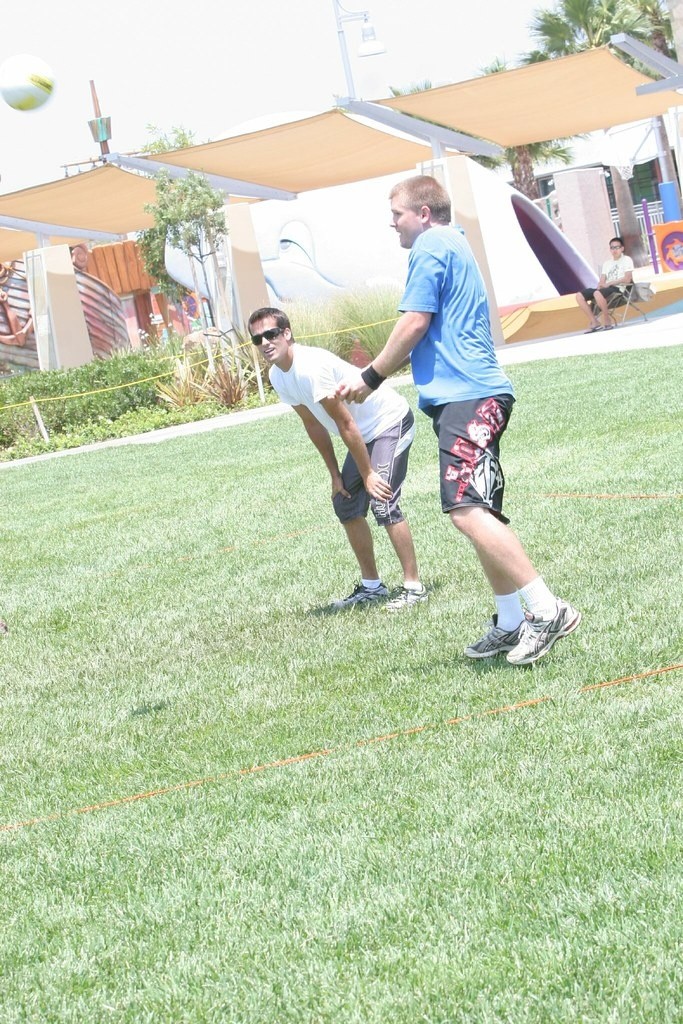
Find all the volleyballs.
[1,51,56,112]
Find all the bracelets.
[361,364,387,390]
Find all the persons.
[247,308,428,613]
[575,238,634,333]
[334,175,581,665]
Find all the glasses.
[251,328,283,347]
[610,246,622,249]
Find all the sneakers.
[382,585,429,613]
[330,579,389,610]
[506,597,581,665]
[465,614,528,658]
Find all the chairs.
[590,277,647,326]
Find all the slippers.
[603,324,614,330]
[584,326,603,334]
